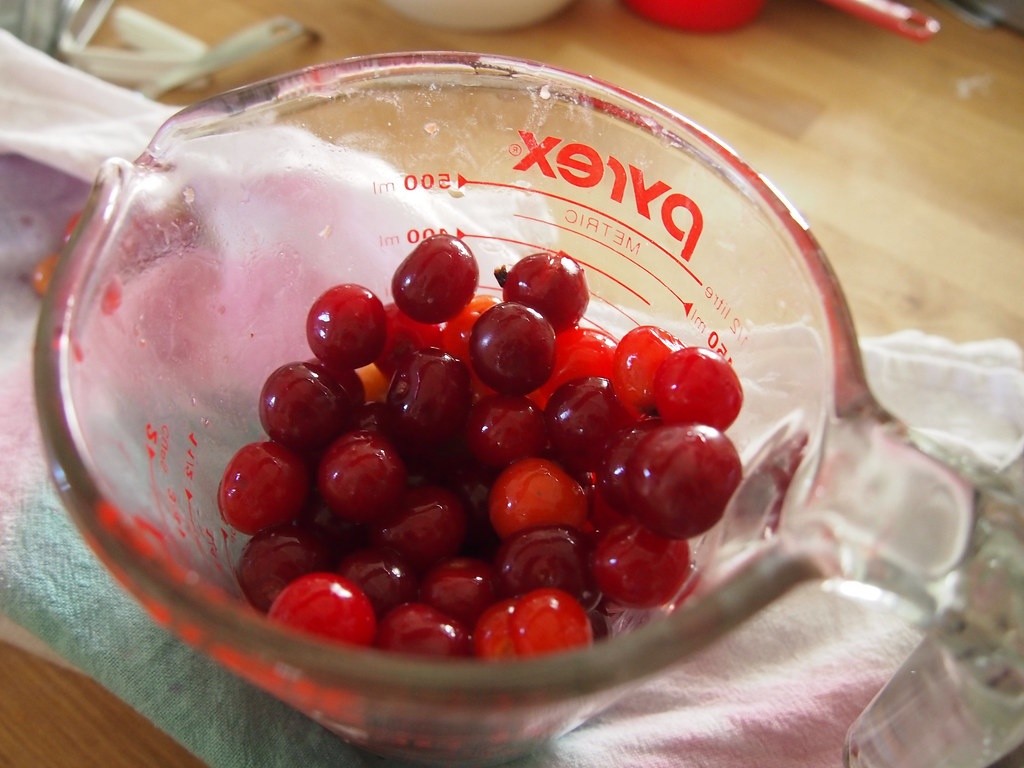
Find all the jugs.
[34,37,1022,767]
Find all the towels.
[0,29,1024,768]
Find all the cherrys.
[215,233,745,662]
